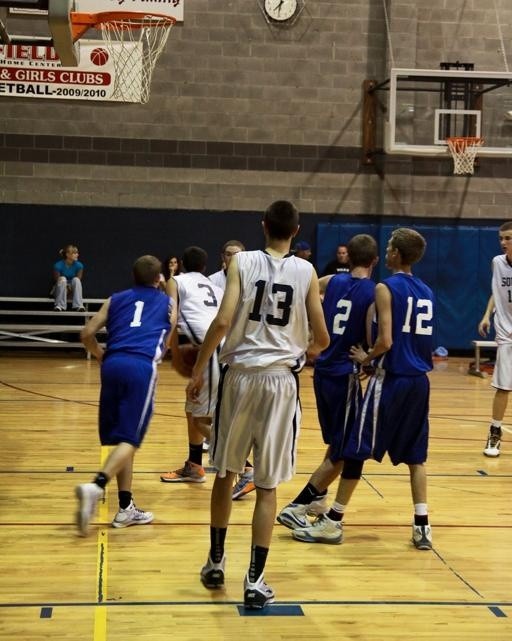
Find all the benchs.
[0,297,191,361]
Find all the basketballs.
[173,344,196,376]
[91,47,109,64]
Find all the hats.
[295,241,311,251]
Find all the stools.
[471,341,499,372]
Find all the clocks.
[264,0,298,22]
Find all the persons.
[165,240,256,502]
[51,245,87,310]
[289,226,436,551]
[71,255,175,539]
[186,201,331,612]
[160,234,379,364]
[479,220,512,456]
[276,234,379,545]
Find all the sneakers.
[232,467,255,500]
[112,501,154,528]
[200,554,225,588]
[160,461,206,483]
[483,433,500,457]
[77,306,85,312]
[74,482,102,536]
[411,524,432,550]
[55,307,61,311]
[277,495,343,545]
[243,572,275,609]
